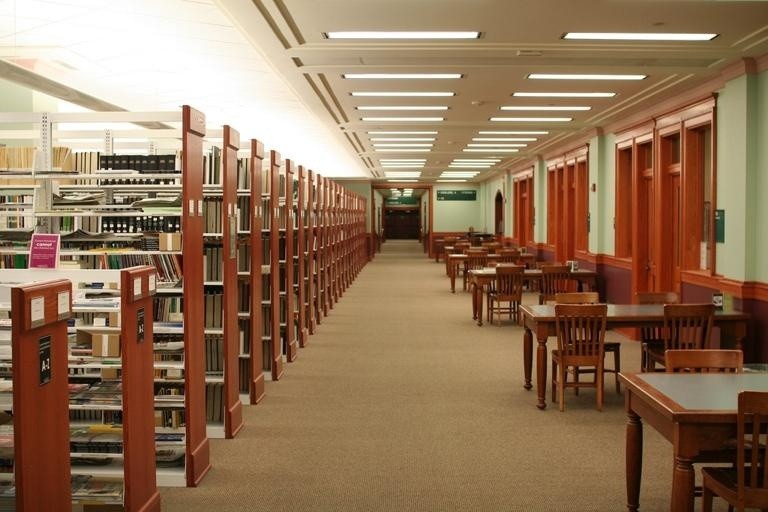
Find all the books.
[206,384,223,423]
[1,180,34,203]
[0,233,32,248]
[1,254,29,270]
[293,233,299,255]
[293,260,298,283]
[262,341,271,370]
[66,380,122,406]
[51,142,183,173]
[2,479,14,497]
[280,299,287,322]
[280,333,287,357]
[239,280,250,312]
[205,290,223,328]
[239,197,249,230]
[70,439,123,453]
[294,289,298,311]
[1,381,13,407]
[0,319,12,363]
[278,204,285,229]
[280,267,286,291]
[239,359,250,393]
[262,236,270,264]
[262,274,270,300]
[154,352,183,363]
[239,318,250,354]
[68,473,122,500]
[262,306,271,336]
[59,256,150,270]
[262,198,269,229]
[54,216,181,251]
[0,424,12,446]
[67,281,122,377]
[155,447,184,468]
[239,237,250,271]
[151,256,182,289]
[237,157,254,189]
[1,147,35,173]
[53,178,182,212]
[205,335,225,372]
[292,207,298,227]
[204,201,223,233]
[154,369,185,383]
[155,432,185,441]
[279,235,285,259]
[294,323,301,344]
[203,247,223,281]
[0,209,32,232]
[203,146,223,185]
[154,295,184,323]
[293,179,299,201]
[154,384,185,429]
[261,169,271,193]
[278,173,286,197]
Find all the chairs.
[635,291,677,370]
[538,266,571,305]
[641,303,711,373]
[555,293,621,395]
[550,304,608,413]
[665,346,743,498]
[453,245,470,254]
[700,388,768,512]
[482,243,500,253]
[467,251,488,294]
[498,250,520,264]
[487,265,524,325]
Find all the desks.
[435,237,469,261]
[444,246,511,277]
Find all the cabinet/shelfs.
[2,104,211,489]
[69,265,163,512]
[205,124,240,439]
[0,279,72,512]
[237,139,368,405]
